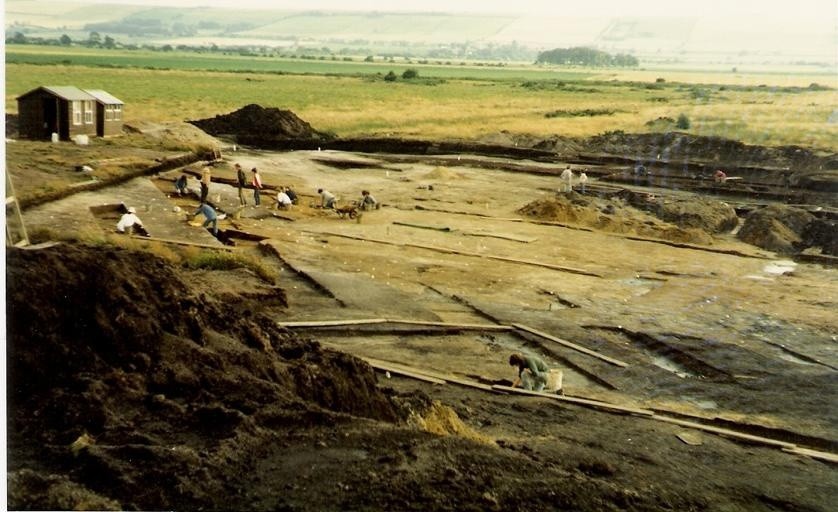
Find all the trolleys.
[332,205,358,220]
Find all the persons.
[194,204,219,235]
[271,186,292,210]
[636,164,649,177]
[358,191,376,207]
[199,163,214,204]
[559,165,574,194]
[713,169,727,184]
[175,175,189,197]
[234,163,248,206]
[116,207,144,235]
[510,353,551,391]
[579,169,589,195]
[284,186,299,205]
[251,167,264,207]
[317,189,337,209]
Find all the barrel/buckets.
[548,369,563,392]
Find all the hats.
[128,207,136,213]
